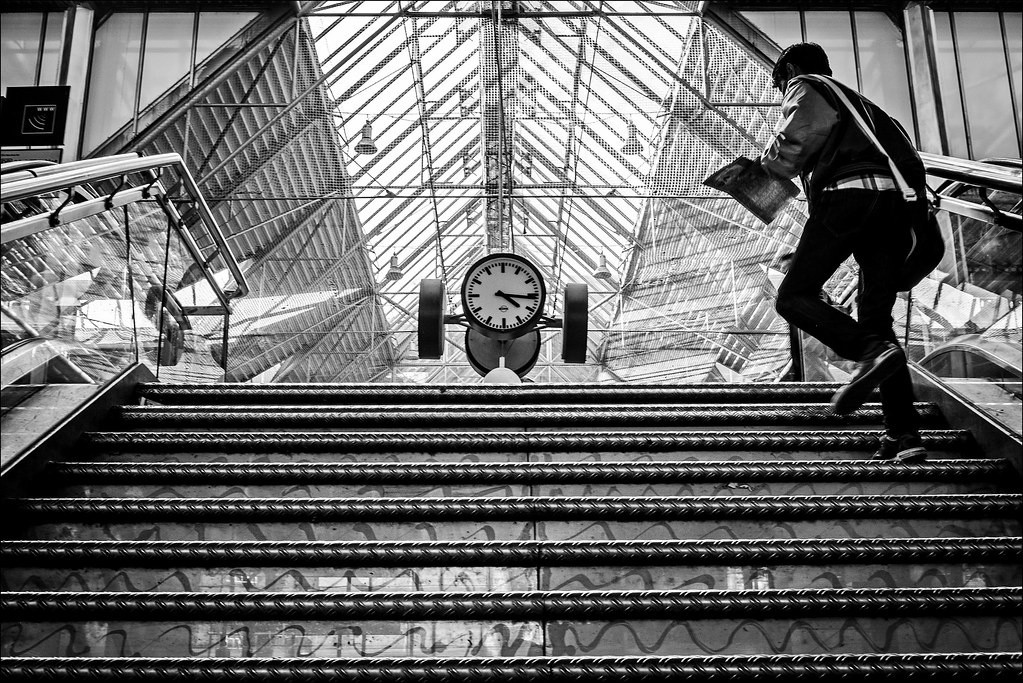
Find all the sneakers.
[831,341,907,413]
[868,434,929,462]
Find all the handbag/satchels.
[897,210,944,294]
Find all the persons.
[756,39,930,461]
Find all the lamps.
[592,255,614,280]
[622,118,643,154]
[355,121,379,154]
[386,251,403,280]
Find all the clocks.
[464,323,543,380]
[462,253,545,338]
[561,282,590,365]
[416,279,448,361]
